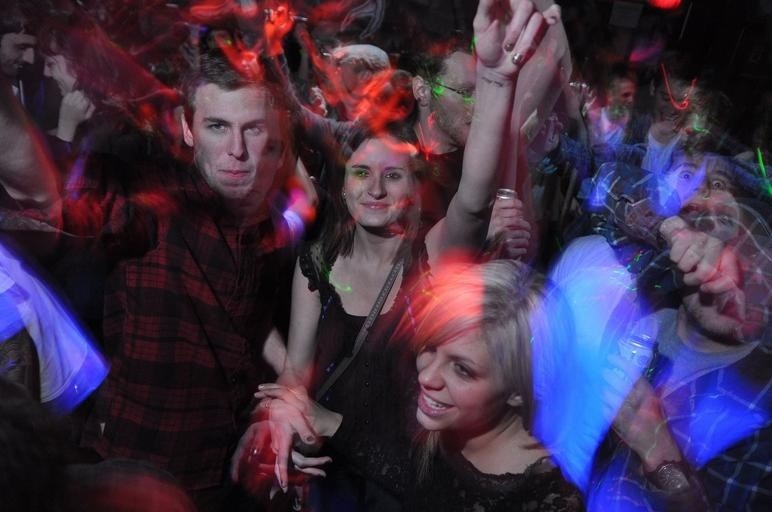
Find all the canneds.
[493,188,519,201]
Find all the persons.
[1,0,772,512]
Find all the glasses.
[433,81,474,98]
